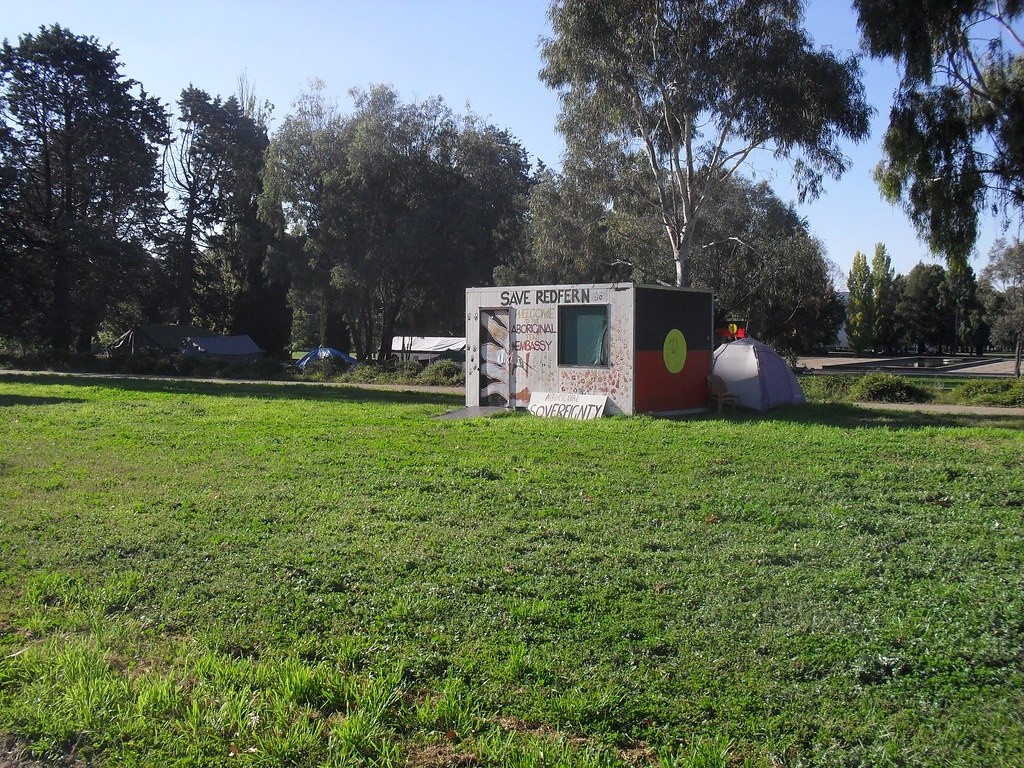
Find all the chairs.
[706,374,738,417]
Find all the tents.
[713,335,805,414]
[106,323,358,373]
